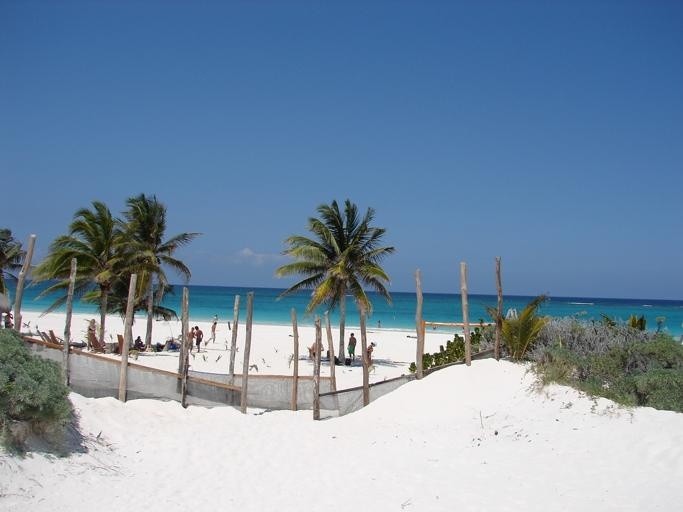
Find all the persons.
[309,341,325,357]
[367,341,376,367]
[187,327,196,353]
[194,326,204,353]
[134,335,147,352]
[347,332,357,362]
[86,319,96,352]
[3,312,14,328]
[207,322,218,344]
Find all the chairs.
[36,329,76,348]
[116,334,139,360]
